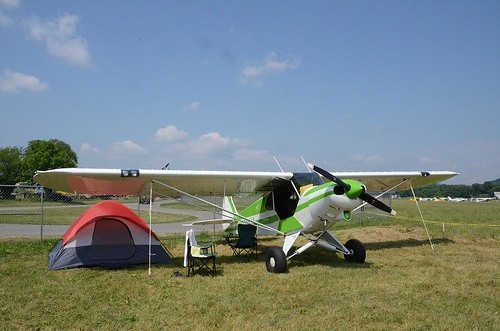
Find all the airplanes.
[33,156,459,277]
[409,196,496,203]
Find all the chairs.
[184,228,219,279]
[225,224,258,261]
